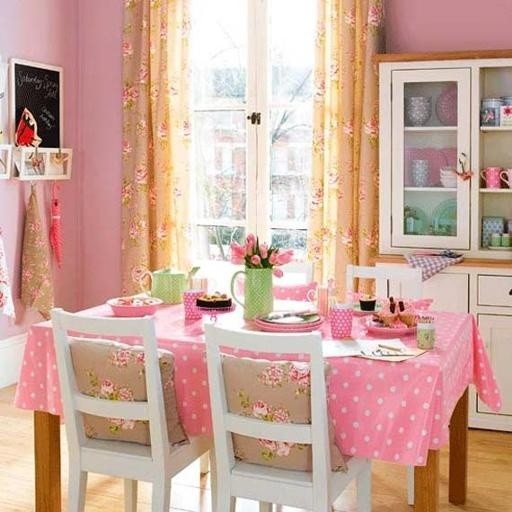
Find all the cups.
[330,305,352,337]
[307,286,336,315]
[230,267,275,320]
[358,299,376,311]
[480,165,500,191]
[183,289,204,320]
[415,323,436,350]
[499,168,512,188]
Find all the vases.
[226,264,279,322]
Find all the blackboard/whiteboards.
[9,58,63,148]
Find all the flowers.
[227,230,295,281]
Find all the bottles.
[489,232,501,245]
[482,94,512,129]
[501,233,510,245]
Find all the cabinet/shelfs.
[371,49,512,260]
[368,248,512,436]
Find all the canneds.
[482,96,512,127]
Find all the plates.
[429,199,456,238]
[487,245,511,251]
[435,89,457,126]
[404,147,456,188]
[366,323,416,338]
[352,302,381,313]
[254,310,324,333]
[404,207,427,234]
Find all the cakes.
[196,291,232,307]
[359,294,376,311]
[374,298,433,329]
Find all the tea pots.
[139,265,201,306]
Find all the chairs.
[346,264,423,305]
[48,307,215,512]
[226,261,316,307]
[197,323,376,512]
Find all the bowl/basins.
[108,297,163,318]
[404,97,432,128]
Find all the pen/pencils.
[378,344,404,353]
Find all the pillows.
[64,332,194,448]
[217,347,351,478]
